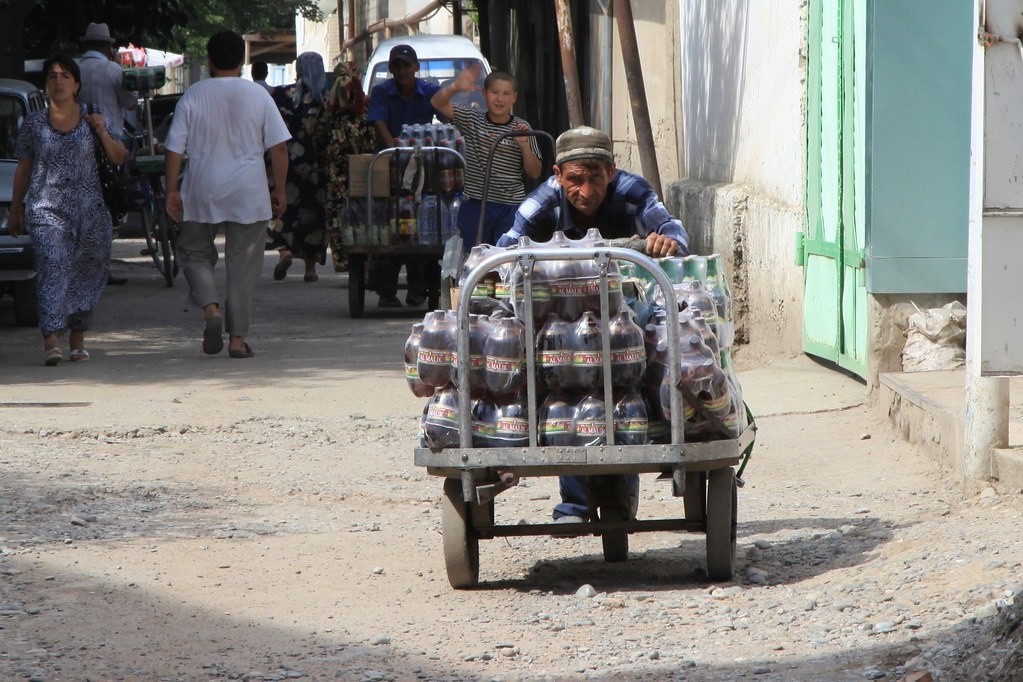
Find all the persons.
[164,30,294,356]
[497,124,689,537]
[250,53,379,293]
[430,61,544,251]
[450,53,487,111]
[73,22,137,284]
[7,53,126,363]
[367,45,444,305]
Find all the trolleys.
[413,247,758,588]
[345,146,468,320]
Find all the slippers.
[203,317,223,354]
[45,347,63,366]
[228,342,255,358]
[69,349,89,362]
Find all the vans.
[363,34,492,116]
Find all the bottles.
[404,226,750,447]
[343,125,478,247]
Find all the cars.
[1,76,47,327]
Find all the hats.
[555,124,613,165]
[80,23,116,43]
[389,45,417,72]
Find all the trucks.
[114,46,185,98]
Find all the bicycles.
[124,113,184,288]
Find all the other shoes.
[305,269,318,281]
[274,250,293,281]
[551,515,587,539]
[378,295,402,307]
[405,289,426,306]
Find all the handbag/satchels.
[87,103,128,227]
